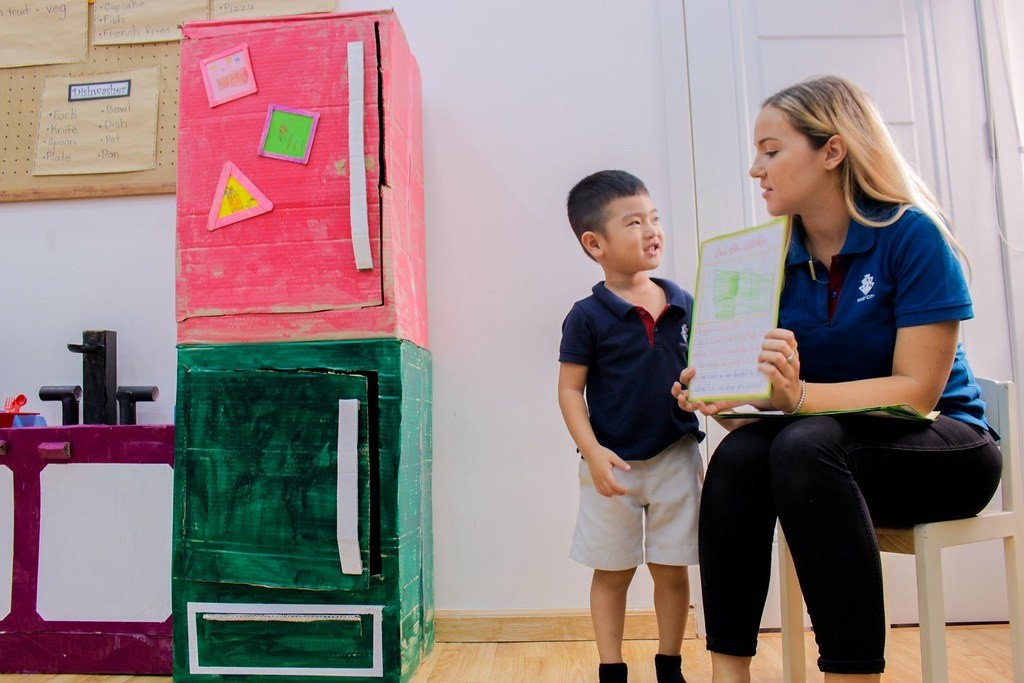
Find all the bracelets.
[783,380,806,415]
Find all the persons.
[557,169,704,683]
[671,76,1003,682]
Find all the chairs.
[778,375,1024,683]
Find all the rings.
[793,341,798,350]
[786,351,795,361]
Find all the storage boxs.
[177,7,430,349]
[0,425,174,675]
[171,338,435,683]
